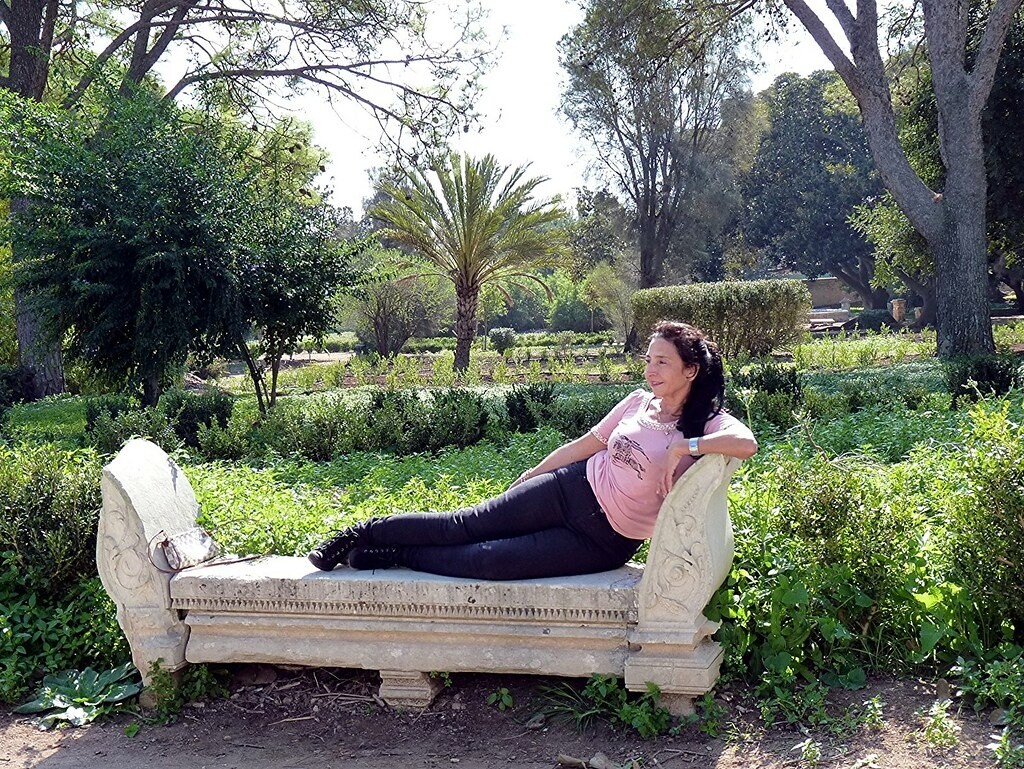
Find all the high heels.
[307,527,361,572]
[347,546,399,572]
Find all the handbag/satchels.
[162,527,220,569]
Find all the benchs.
[92,439,734,715]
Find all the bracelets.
[520,469,529,482]
[689,437,701,457]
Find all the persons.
[306,321,760,581]
